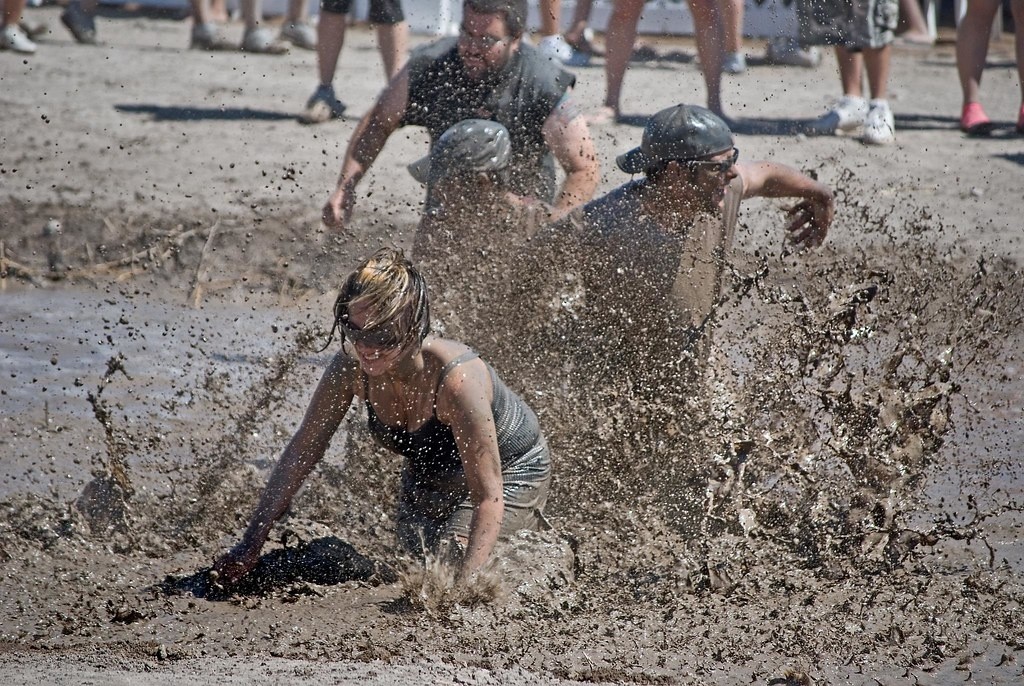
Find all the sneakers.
[862,99,894,144]
[805,94,871,135]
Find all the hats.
[407,119,511,185]
[616,103,734,174]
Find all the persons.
[210,245,551,593]
[322,0,600,263]
[504,105,833,412]
[407,114,572,320]
[0,0,1024,146]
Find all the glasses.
[459,28,513,52]
[338,319,401,349]
[686,148,739,173]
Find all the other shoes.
[241,29,292,54]
[190,22,235,51]
[539,35,591,66]
[299,85,346,122]
[4,26,39,55]
[280,24,318,49]
[723,51,747,75]
[768,42,816,68]
[1016,105,1024,132]
[959,103,993,133]
